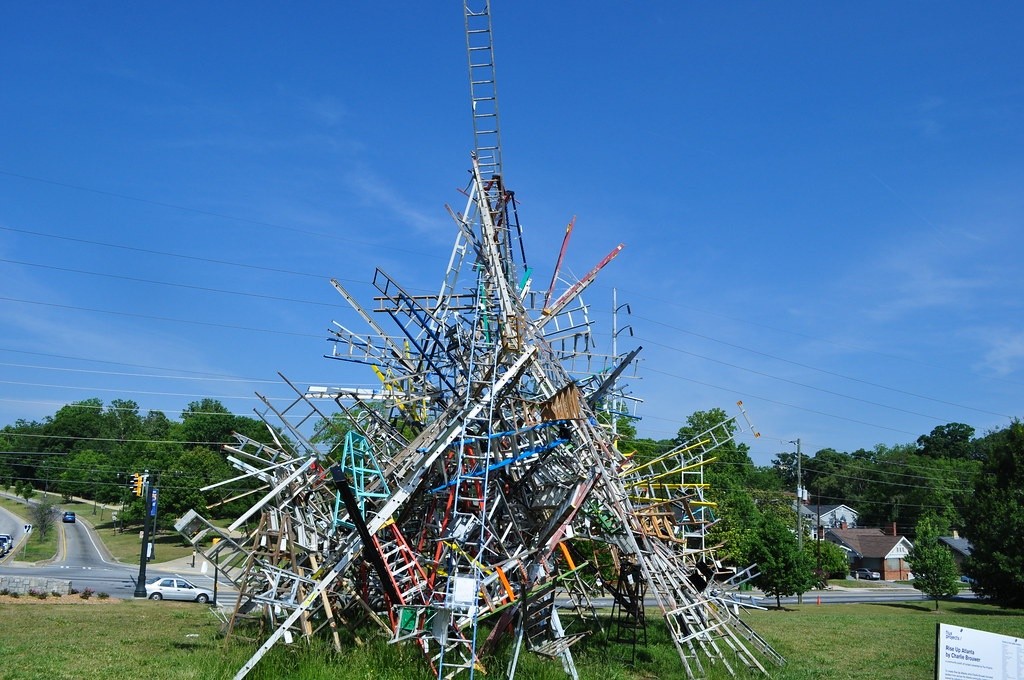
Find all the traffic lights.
[132,476,143,497]
[129,473,138,491]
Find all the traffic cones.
[817,596,821,605]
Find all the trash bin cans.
[740,583,752,591]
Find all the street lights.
[789,437,802,603]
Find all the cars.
[960,575,975,584]
[850,567,880,580]
[145,575,214,604]
[63,511,76,523]
[0,534,14,557]
